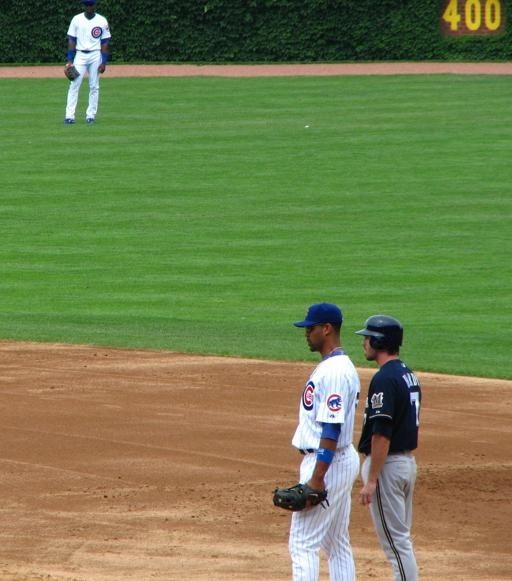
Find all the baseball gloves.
[64,64,80,81]
[272,485,327,511]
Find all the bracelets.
[102,51,109,65]
[66,50,76,62]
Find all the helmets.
[355,313,403,344]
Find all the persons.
[284,302,362,581]
[63,1,112,128]
[354,315,424,581]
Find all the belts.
[298,448,316,454]
[76,49,94,54]
[365,450,411,456]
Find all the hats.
[292,302,344,328]
[82,0,96,6]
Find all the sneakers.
[64,119,75,124]
[87,118,94,124]
